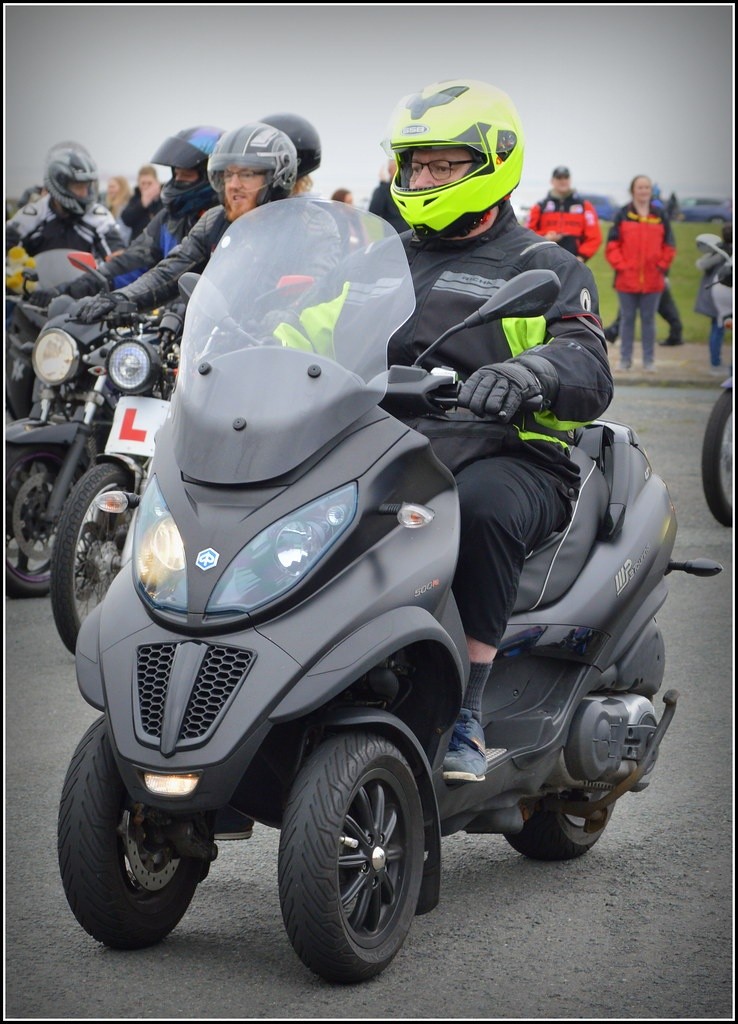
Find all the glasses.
[404,160,475,181]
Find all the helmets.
[390,80,522,235]
[44,141,101,215]
[213,123,297,206]
[150,127,223,218]
[262,115,321,179]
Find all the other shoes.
[667,321,682,345]
[605,329,617,342]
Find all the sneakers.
[442,708,485,782]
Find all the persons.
[331,159,410,254]
[605,175,683,373]
[528,166,602,263]
[7,112,344,351]
[695,226,732,376]
[215,78,612,782]
[666,194,676,217]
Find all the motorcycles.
[53,196,721,985]
[5,203,138,599]
[694,233,732,527]
[50,254,201,654]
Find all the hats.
[554,167,569,177]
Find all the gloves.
[70,294,127,322]
[29,288,60,306]
[457,363,540,422]
